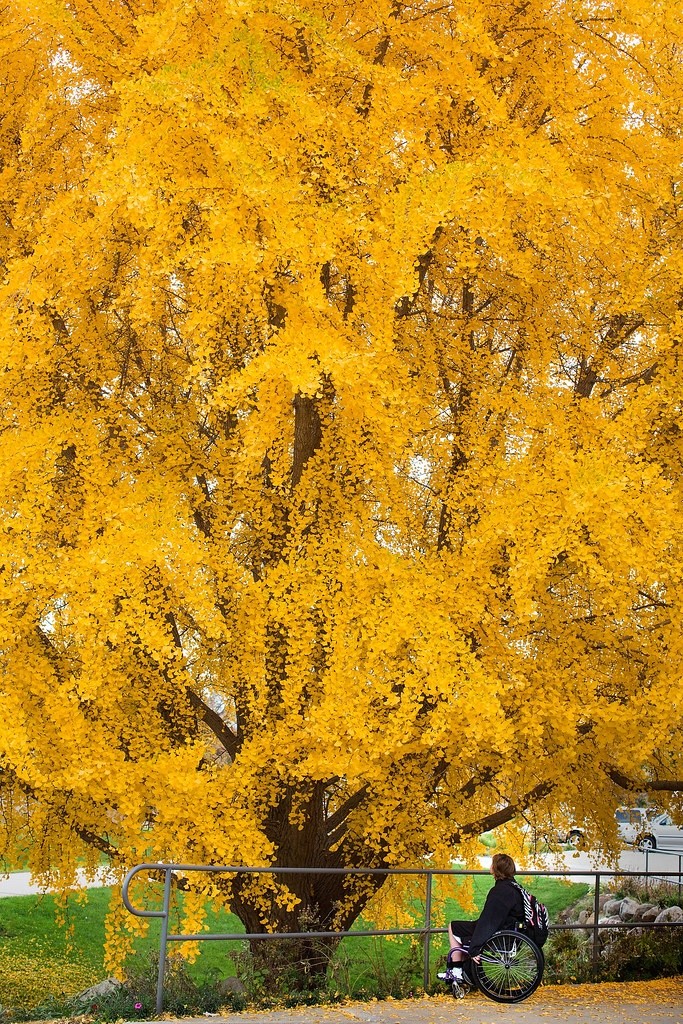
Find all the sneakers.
[436,967,464,981]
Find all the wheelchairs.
[444,921,545,1003]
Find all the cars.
[556,806,683,853]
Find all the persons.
[437,854,525,980]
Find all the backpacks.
[511,881,549,948]
